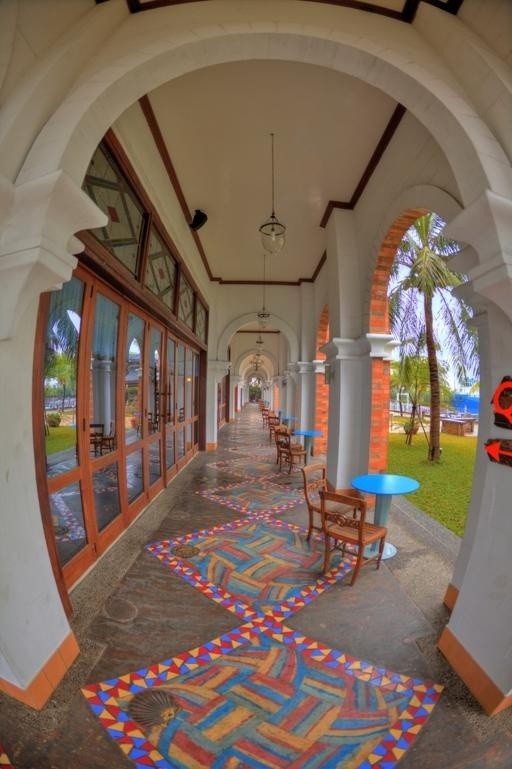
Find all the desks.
[291,429,324,470]
[351,474,421,560]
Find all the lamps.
[259,132,286,254]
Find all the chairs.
[261,407,307,475]
[298,461,388,588]
